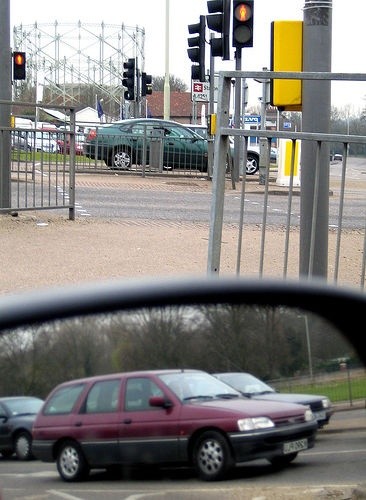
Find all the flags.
[97,95,104,118]
[147,106,153,118]
[119,103,125,119]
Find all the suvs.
[30,367,320,480]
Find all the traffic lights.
[187,14,207,83]
[122,57,135,101]
[231,0,255,49]
[141,72,152,96]
[13,50,26,80]
[206,0,230,60]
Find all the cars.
[211,368,334,430]
[82,118,232,175]
[11,125,86,155]
[0,393,54,461]
[182,123,260,175]
